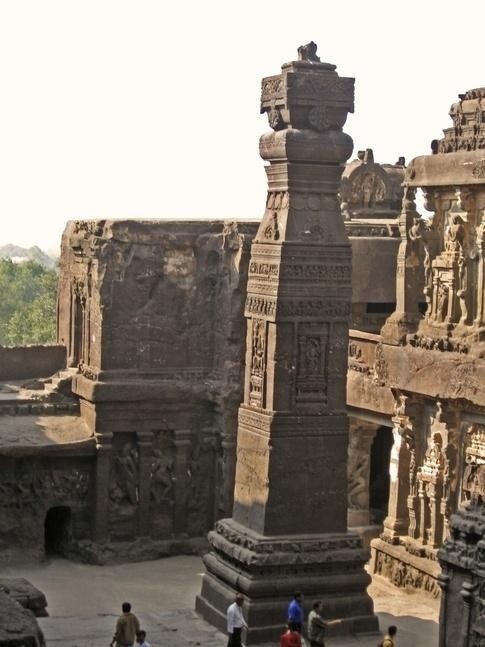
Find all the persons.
[280,622,307,647]
[407,213,485,296]
[136,630,151,647]
[110,601,140,647]
[307,599,346,647]
[111,441,213,505]
[383,626,397,647]
[226,592,248,647]
[287,592,305,634]
